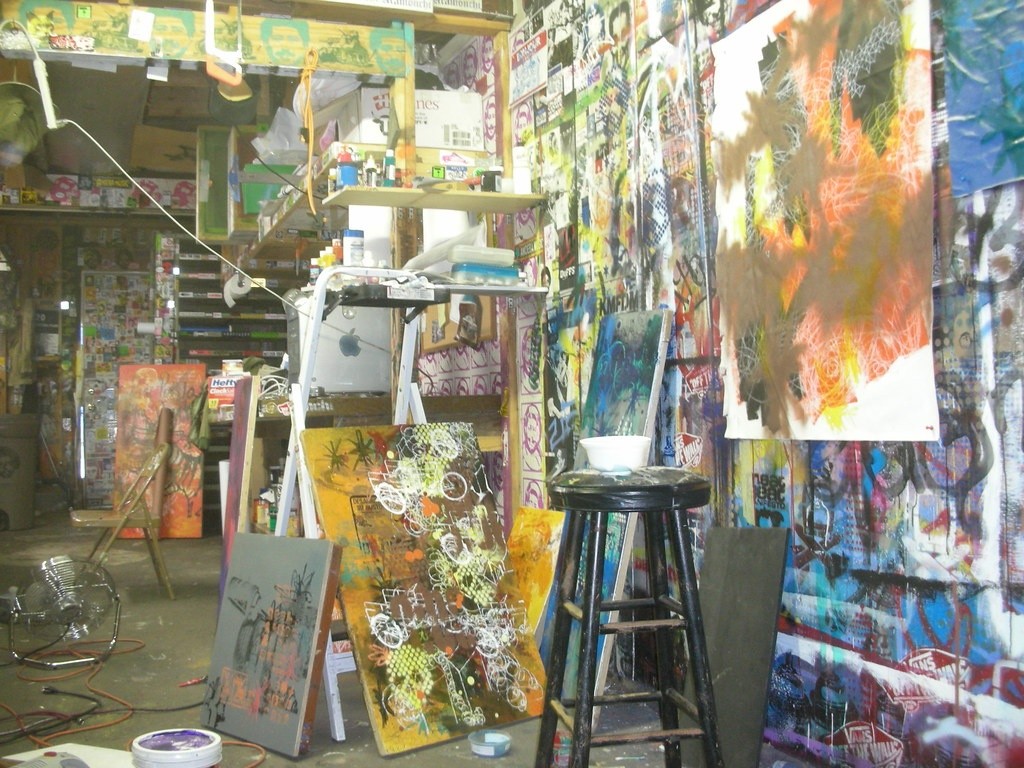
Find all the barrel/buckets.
[131,728,224,768]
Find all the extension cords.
[33,58,59,130]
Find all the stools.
[535,466,726,768]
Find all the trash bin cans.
[0,411,45,532]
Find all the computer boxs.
[284,290,397,397]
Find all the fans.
[6,553,122,670]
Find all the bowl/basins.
[469,730,513,757]
[580,435,653,473]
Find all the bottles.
[326,147,398,189]
[308,239,343,286]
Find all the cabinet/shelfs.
[199,140,552,539]
[170,233,289,513]
[221,376,520,644]
[33,302,62,393]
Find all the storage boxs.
[447,243,515,267]
[310,84,485,151]
[451,262,518,286]
[242,164,297,216]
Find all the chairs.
[70,442,176,600]
